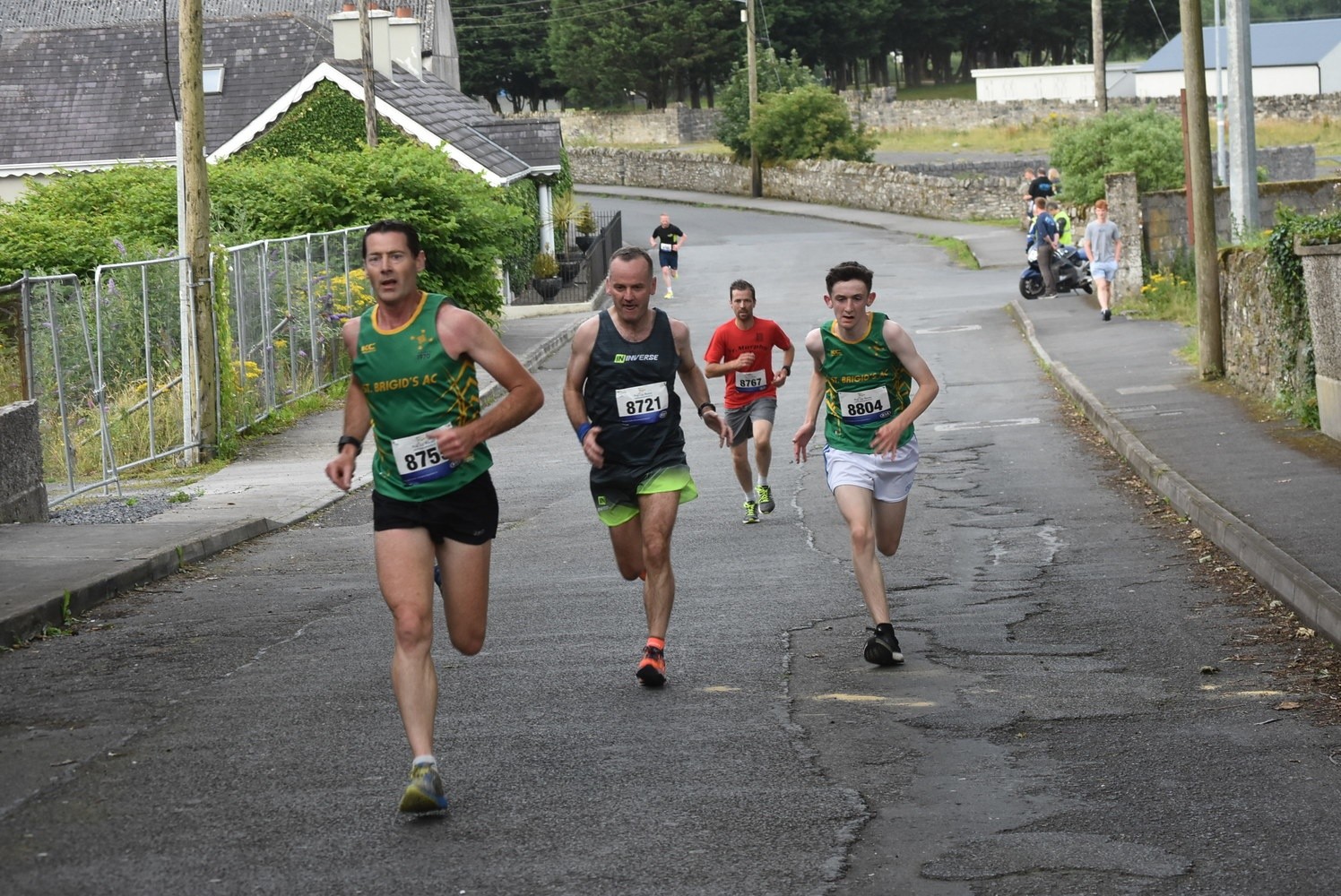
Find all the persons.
[651,213,687,300]
[1017,166,1122,322]
[563,245,734,687]
[324,219,544,816]
[703,279,795,525]
[792,262,939,665]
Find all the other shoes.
[1038,292,1058,300]
[1101,309,1111,321]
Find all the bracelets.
[578,423,592,444]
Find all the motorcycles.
[1018,236,1093,300]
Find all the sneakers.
[755,484,775,513]
[669,266,678,279]
[664,292,674,299]
[402,761,448,813]
[864,625,905,665]
[743,500,760,523]
[636,646,666,686]
[638,568,647,580]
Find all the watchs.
[337,436,362,457]
[782,366,791,377]
[698,402,716,417]
